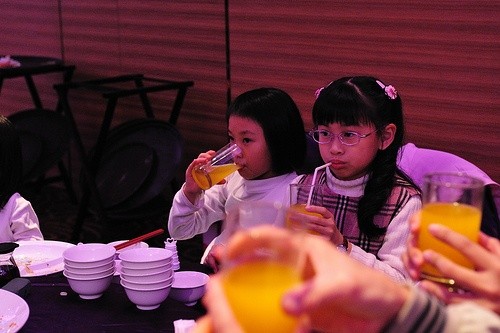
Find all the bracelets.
[337,236,348,249]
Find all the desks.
[18,255,215,333]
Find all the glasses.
[309,125,389,146]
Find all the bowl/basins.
[118,247,174,310]
[64,243,117,299]
[108,240,149,261]
[170,271,210,306]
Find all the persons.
[285,76,424,282]
[0,116,45,261]
[168,88,319,273]
[217,210,500,333]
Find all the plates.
[0,288,30,333]
[0,240,77,278]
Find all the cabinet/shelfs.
[0,56,194,242]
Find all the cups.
[418,171,483,291]
[289,184,325,235]
[218,204,315,333]
[192,142,246,190]
